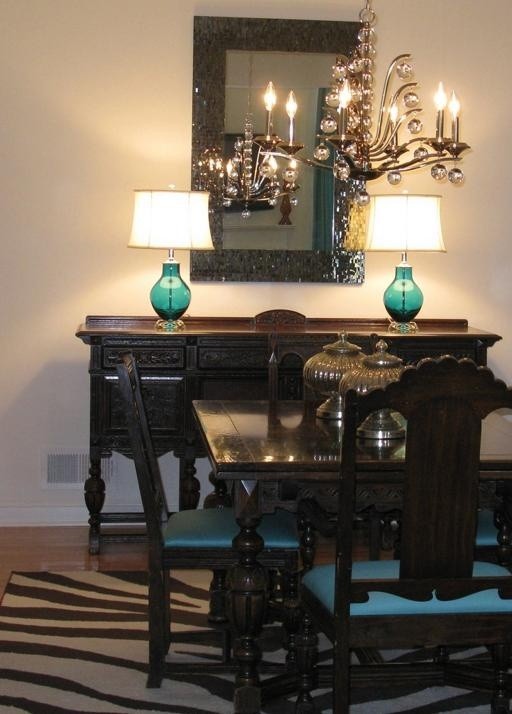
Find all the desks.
[189,396,512,711]
[76,310,502,554]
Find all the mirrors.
[190,16,368,286]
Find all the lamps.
[129,188,217,332]
[196,134,295,216]
[367,195,447,334]
[250,2,470,188]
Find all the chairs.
[291,354,512,713]
[117,356,308,689]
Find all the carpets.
[1,568,512,712]
